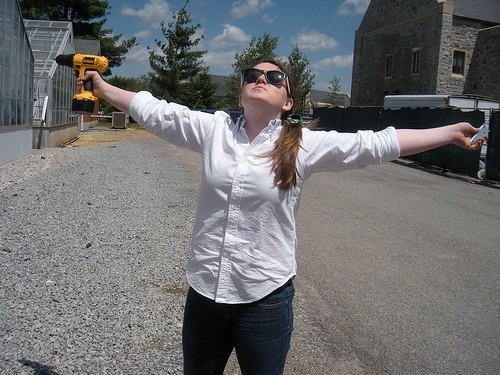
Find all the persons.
[76,59,489,375]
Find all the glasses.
[240,68,291,94]
